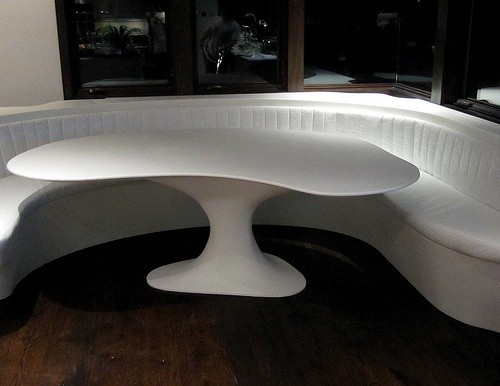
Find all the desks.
[4,127,419,297]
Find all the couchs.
[0,102,499,335]
